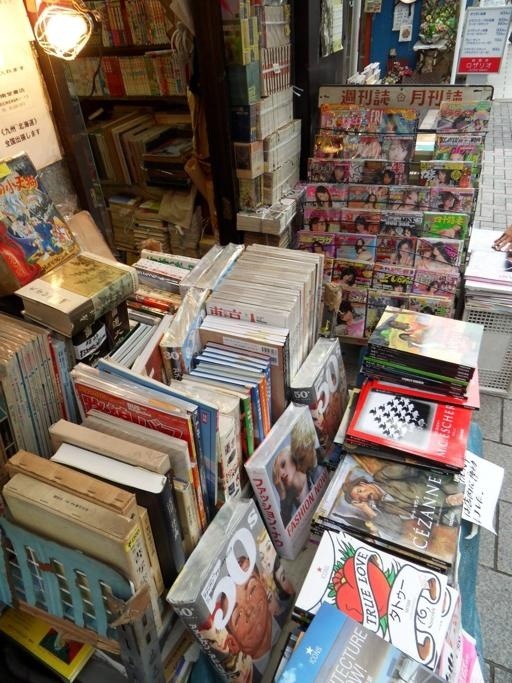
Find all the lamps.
[30,2,103,63]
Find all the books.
[0,1,511,682]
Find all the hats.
[340,475,368,504]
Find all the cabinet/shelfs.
[75,2,300,263]
